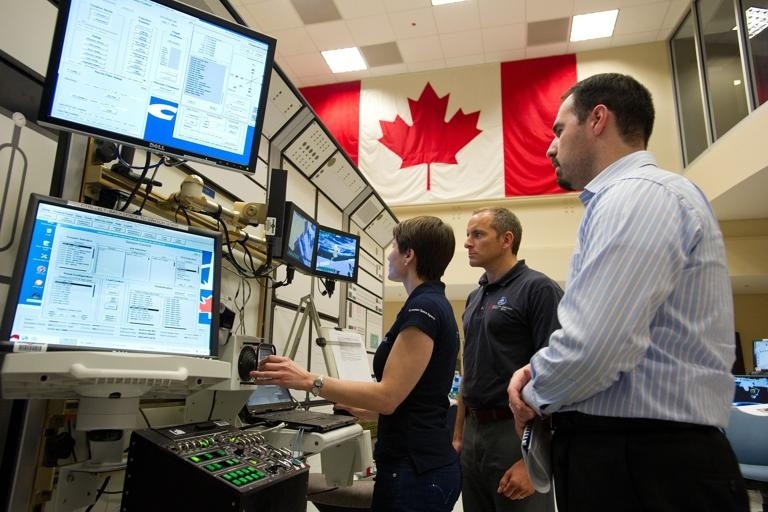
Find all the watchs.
[311,374,325,397]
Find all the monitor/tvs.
[280,201,320,275]
[37,0,277,175]
[752,338,768,372]
[310,225,360,283]
[0,193,223,360]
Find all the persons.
[250,215,463,512]
[452,207,565,512]
[507,73,750,512]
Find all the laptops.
[243,347,356,433]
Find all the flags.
[298,53,585,207]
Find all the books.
[521,418,552,495]
